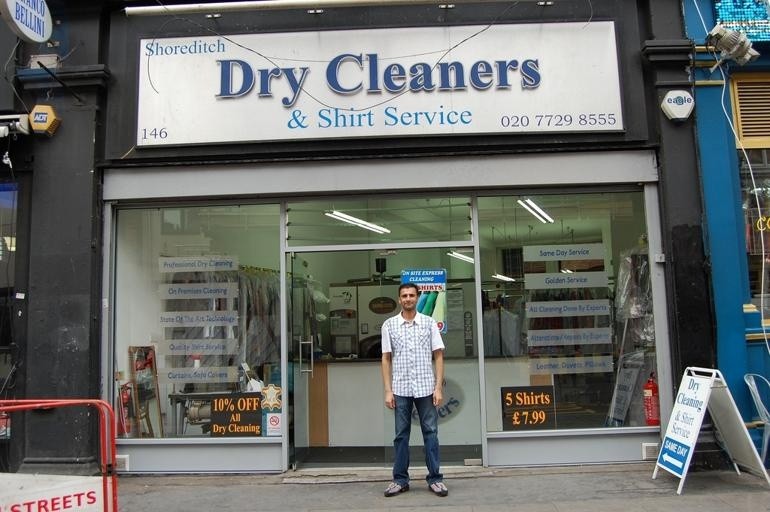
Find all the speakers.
[376,258,386,273]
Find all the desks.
[166,389,280,437]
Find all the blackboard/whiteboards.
[129,345,159,404]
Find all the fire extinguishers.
[117,381,133,434]
[642,372,660,426]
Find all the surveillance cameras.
[708,24,760,66]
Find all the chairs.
[744,373,770,470]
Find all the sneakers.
[383,482,408,496]
[429,482,448,496]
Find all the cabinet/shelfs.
[328,281,359,359]
[417,277,480,359]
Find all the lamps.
[487,225,516,284]
[557,218,577,276]
[322,204,392,236]
[517,194,554,224]
[444,196,475,265]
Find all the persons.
[380,283,449,497]
